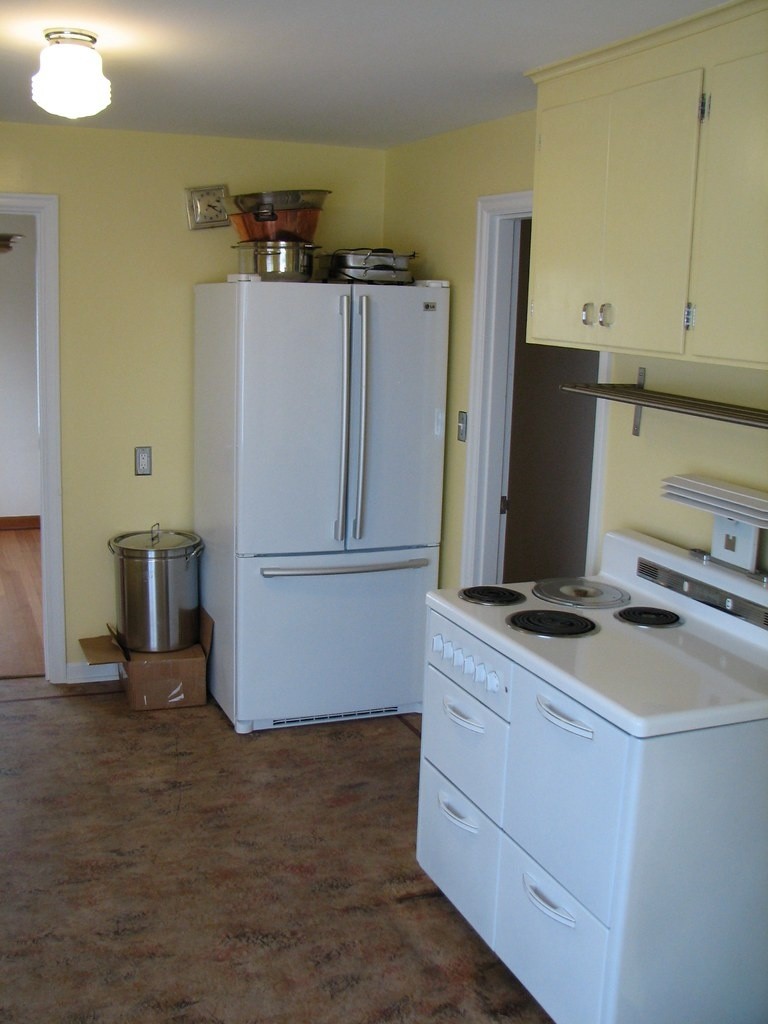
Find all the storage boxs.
[78,604,215,711]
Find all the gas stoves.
[423,526,768,740]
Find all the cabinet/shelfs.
[414,663,509,951]
[526,67,705,360]
[690,51,768,368]
[491,664,768,1023]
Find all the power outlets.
[135,446,152,476]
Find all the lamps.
[31,27,112,120]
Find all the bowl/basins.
[223,189,334,209]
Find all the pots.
[230,240,323,281]
[108,524,204,653]
[227,209,323,241]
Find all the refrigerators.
[192,279,452,736]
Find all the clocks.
[185,184,232,232]
